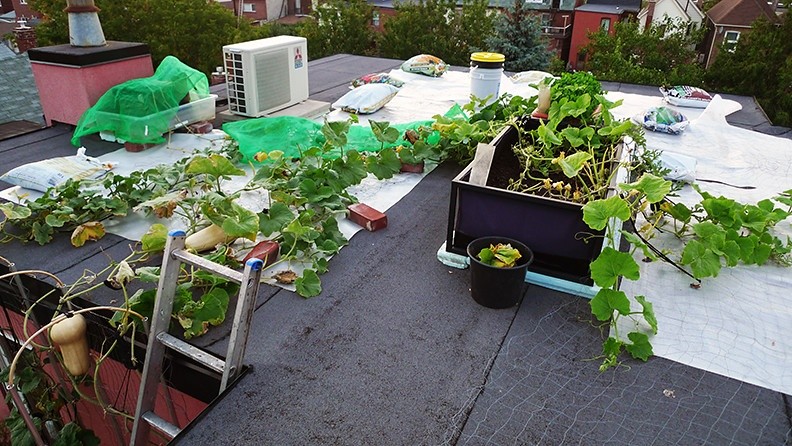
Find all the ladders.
[128,228,263,446]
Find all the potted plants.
[404,71,792,371]
[467,236,533,310]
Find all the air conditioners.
[223,35,309,118]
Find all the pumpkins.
[51,85,551,376]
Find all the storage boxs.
[94,93,218,140]
